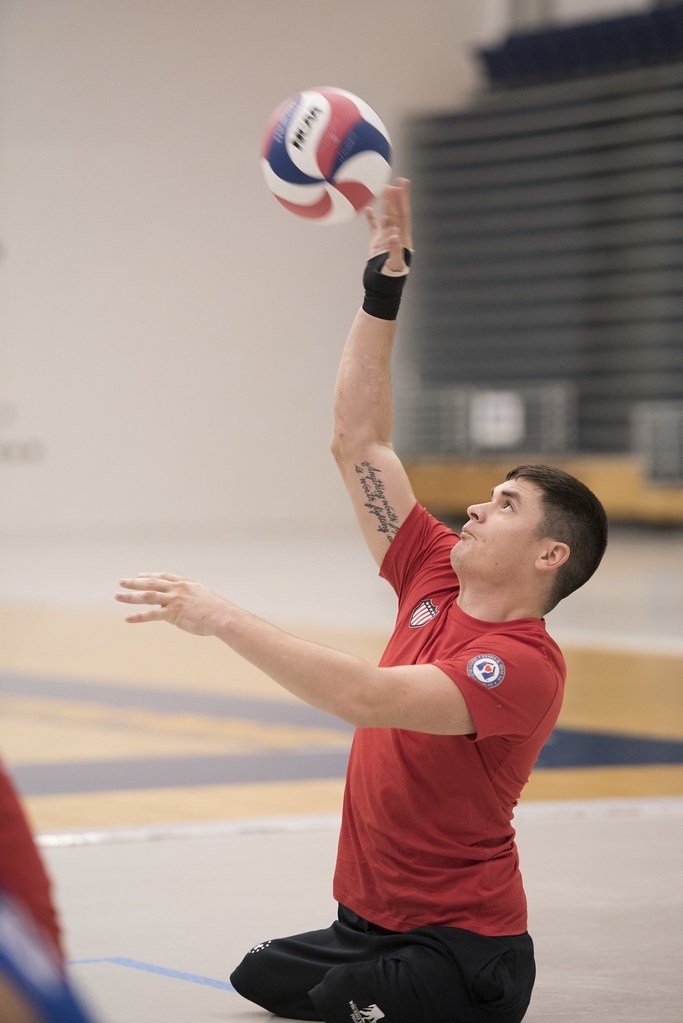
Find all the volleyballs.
[261,86,395,224]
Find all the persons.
[114,175,613,1023]
[0,776,81,1023]
